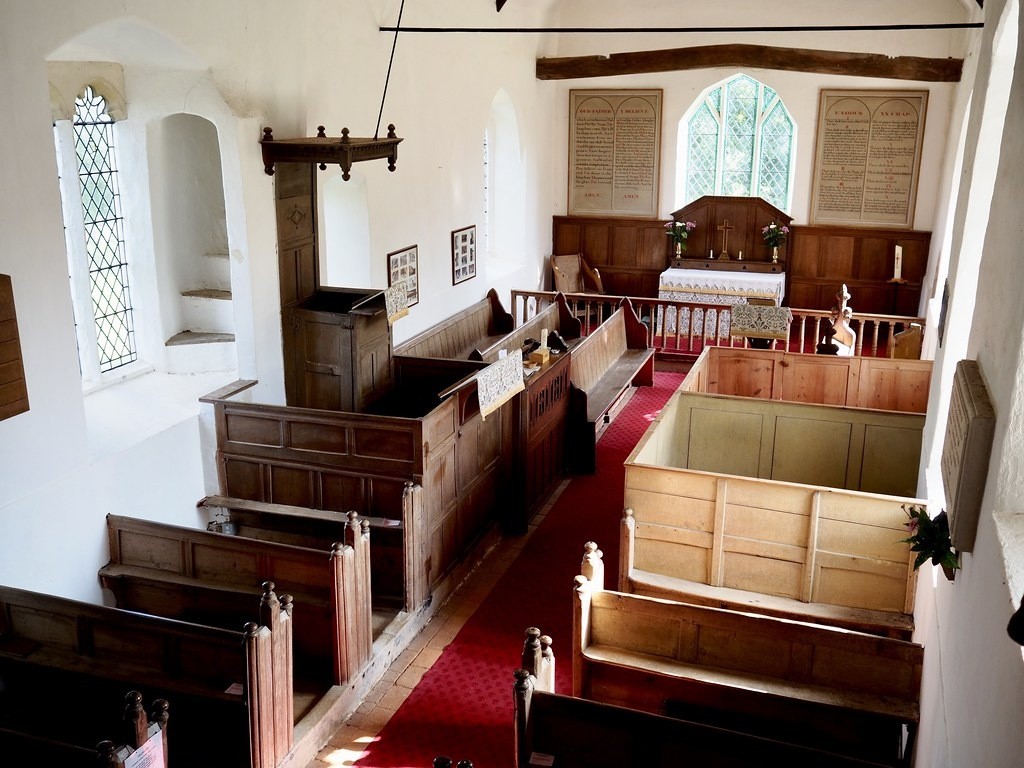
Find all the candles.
[738,251,742,260]
[710,250,713,258]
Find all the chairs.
[891,323,921,360]
[549,253,604,316]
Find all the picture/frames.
[451,225,476,287]
[387,244,419,307]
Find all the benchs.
[394,287,656,476]
[512,506,925,768]
[0,480,429,768]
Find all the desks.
[656,266,786,343]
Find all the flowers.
[761,221,789,250]
[897,503,962,572]
[663,221,696,252]
